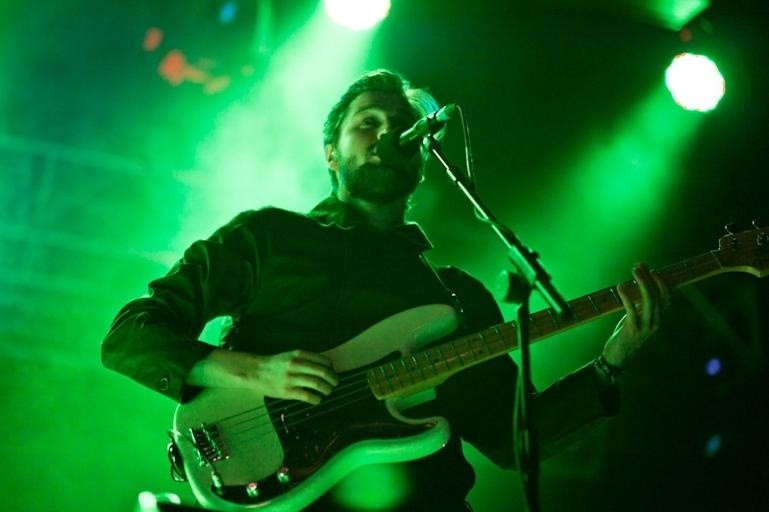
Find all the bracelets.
[595,356,621,386]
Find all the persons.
[98,70,675,510]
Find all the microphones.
[398,102,457,152]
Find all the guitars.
[165,217,769,512]
